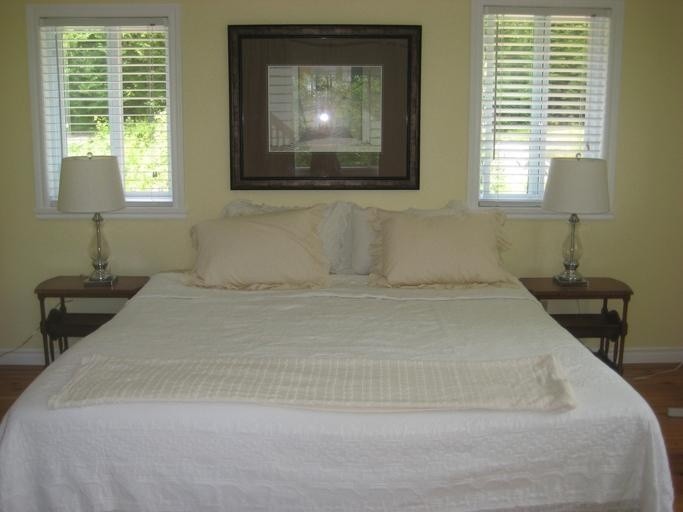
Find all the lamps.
[55,151,126,285]
[541,153,611,285]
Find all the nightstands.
[31,275,150,368]
[519,274,634,378]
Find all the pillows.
[189,203,510,290]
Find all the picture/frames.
[224,22,423,190]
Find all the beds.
[7,268,660,510]
